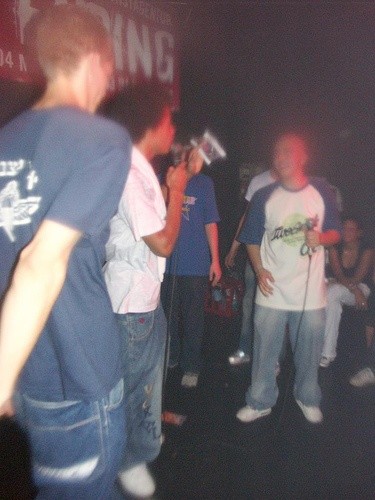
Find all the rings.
[362,303,364,304]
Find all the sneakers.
[349,367,375,387]
[229,350,249,365]
[320,356,335,367]
[295,398,323,424]
[119,461,156,499]
[236,404,271,423]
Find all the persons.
[349,291,375,386]
[224,163,278,364]
[0,5,127,499]
[320,213,373,369]
[236,130,341,423]
[97,86,203,499]
[154,124,224,391]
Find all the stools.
[336,307,367,361]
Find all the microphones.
[306,218,316,259]
[169,143,184,168]
